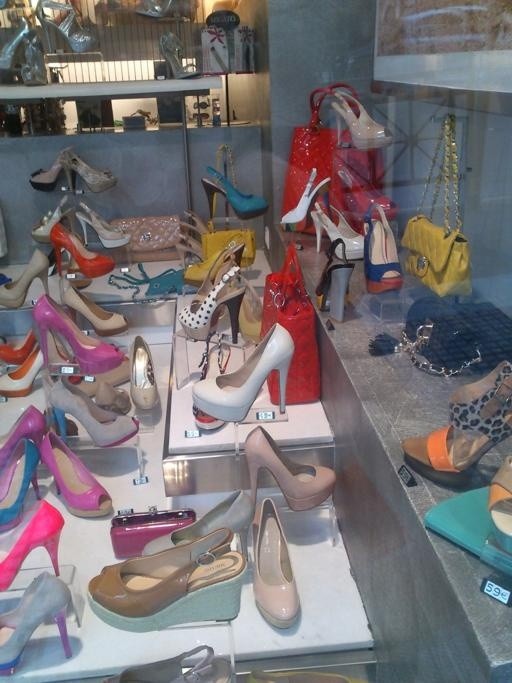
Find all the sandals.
[404,360,512,552]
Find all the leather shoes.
[132,334,159,411]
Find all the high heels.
[159,32,201,79]
[110,509,196,560]
[191,324,294,429]
[1,0,99,86]
[135,0,175,16]
[141,490,254,563]
[331,90,391,151]
[0,501,65,590]
[0,249,140,534]
[29,147,130,291]
[0,571,73,675]
[245,426,336,511]
[85,526,247,633]
[253,498,301,629]
[280,155,404,322]
[177,165,272,341]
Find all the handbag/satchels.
[367,296,511,376]
[402,214,475,297]
[146,267,197,297]
[112,216,183,265]
[260,270,323,403]
[285,83,384,236]
